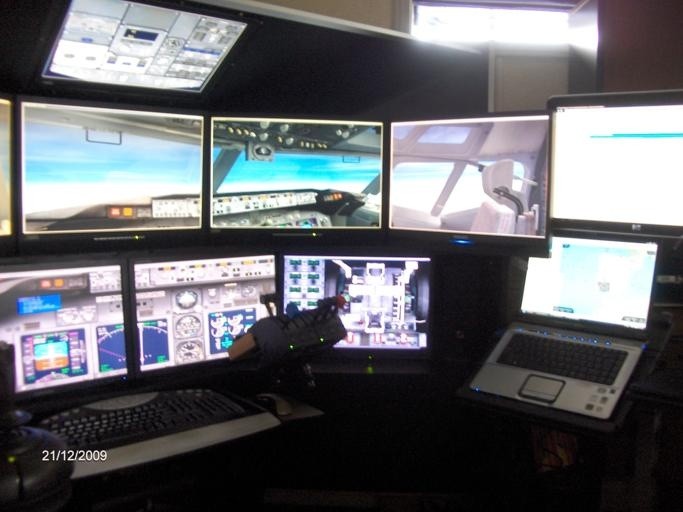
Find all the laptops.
[467,228,663,421]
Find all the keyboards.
[34,382,281,480]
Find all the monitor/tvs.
[281,253,436,355]
[207,113,385,245]
[127,248,280,378]
[31,0,252,100]
[547,88,683,240]
[12,96,207,243]
[0,93,13,244]
[384,108,552,259]
[2,253,131,401]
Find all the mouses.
[253,392,293,416]
